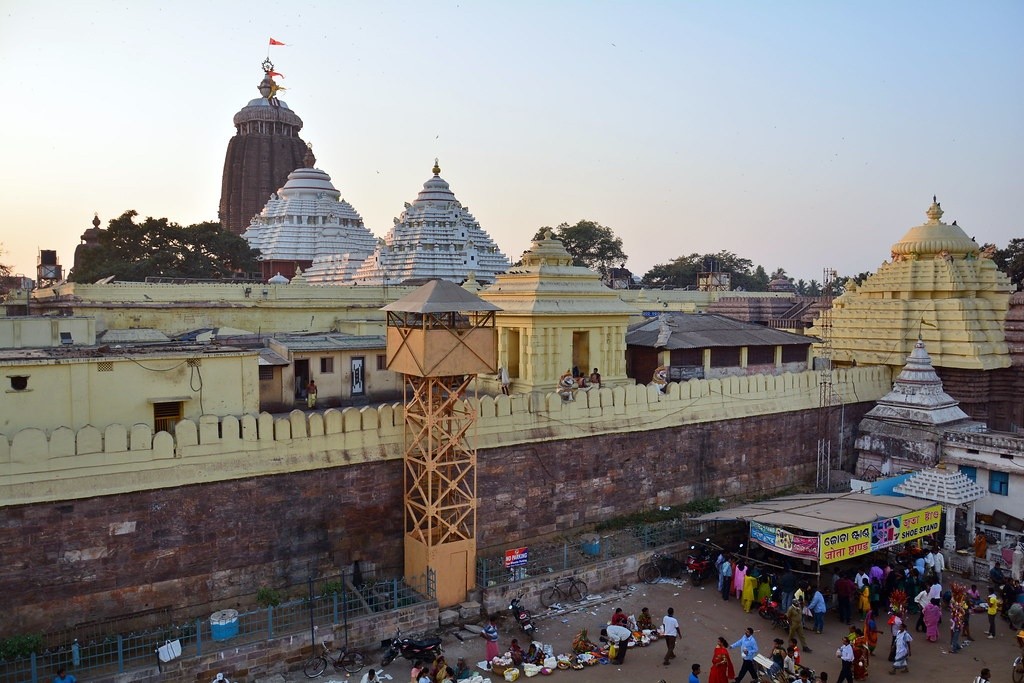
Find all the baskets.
[492,660,514,676]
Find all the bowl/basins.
[558,663,569,670]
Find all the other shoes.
[987,635,995,639]
[847,622,852,625]
[487,663,491,669]
[810,628,817,631]
[902,669,909,673]
[664,661,670,665]
[803,646,812,652]
[984,631,990,634]
[612,661,622,665]
[669,655,676,659]
[816,631,820,634]
[750,680,758,683]
[967,637,975,641]
[870,653,877,657]
[889,670,896,674]
[962,633,968,637]
[735,677,741,683]
[859,619,865,622]
[803,626,808,630]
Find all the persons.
[498,368,509,396]
[213,673,228,682]
[709,530,1024,683]
[689,664,701,683]
[480,616,499,661]
[360,669,380,683]
[572,627,598,652]
[557,368,601,401]
[306,379,318,409]
[636,607,652,629]
[508,639,545,666]
[411,655,469,683]
[612,608,634,628]
[663,607,682,666]
[601,624,632,665]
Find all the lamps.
[52,288,59,295]
[246,288,251,294]
[60,332,74,346]
[663,302,667,306]
[263,289,267,293]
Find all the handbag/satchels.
[887,614,896,625]
[802,606,814,618]
[609,643,616,659]
[836,647,842,658]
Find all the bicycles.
[1010,630,1024,683]
[540,575,588,609]
[303,640,366,679]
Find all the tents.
[689,491,942,586]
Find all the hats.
[792,599,800,605]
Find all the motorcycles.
[636,538,745,588]
[381,627,445,668]
[507,589,538,637]
[758,586,790,634]
